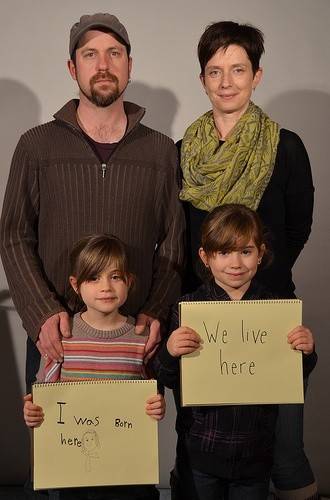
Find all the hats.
[69,13,131,56]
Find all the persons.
[175,21,320,500]
[22,233,166,499]
[146,204,317,499]
[0,14,185,500]
